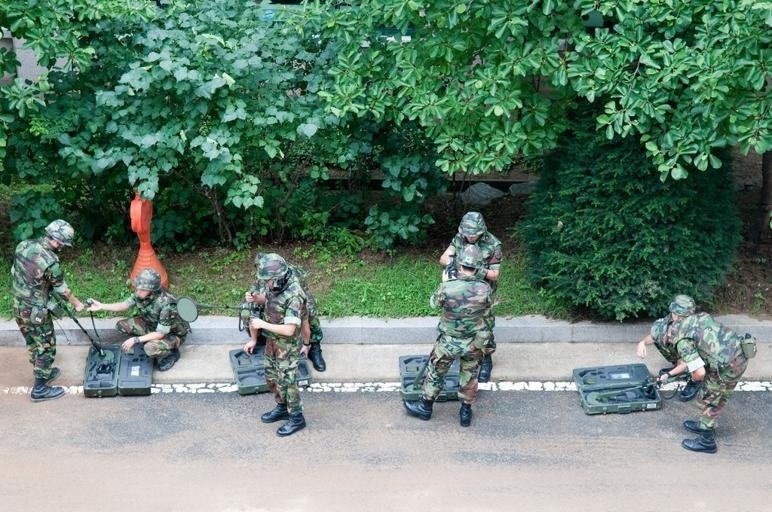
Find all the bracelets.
[136,337,140,343]
[303,340,310,346]
[666,372,671,377]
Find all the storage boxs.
[86,342,155,397]
[230,345,313,396]
[398,355,466,402]
[573,363,663,415]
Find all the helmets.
[457,244,483,269]
[132,267,162,292]
[257,253,289,280]
[253,252,264,266]
[458,212,487,238]
[44,219,74,248]
[669,296,697,318]
[650,318,673,348]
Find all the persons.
[439,211,504,382]
[637,294,702,402]
[403,244,493,427]
[10,219,85,403]
[86,269,190,371]
[650,312,748,453]
[244,253,306,436]
[238,252,325,371]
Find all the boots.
[478,355,493,382]
[308,341,325,371]
[684,420,716,438]
[43,367,60,384]
[30,377,64,402]
[276,413,306,437]
[157,349,180,372]
[682,430,717,453]
[680,379,700,402]
[460,403,473,426]
[404,398,432,420]
[260,402,289,423]
[659,367,675,374]
